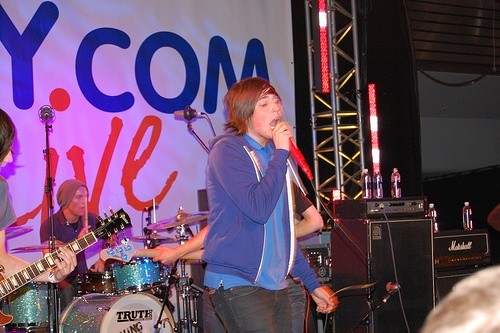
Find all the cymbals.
[147,210,210,231]
[130,235,175,243]
[11,239,65,251]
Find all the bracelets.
[49,269,59,283]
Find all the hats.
[55,179,90,210]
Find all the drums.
[1,282,62,329]
[77,271,116,295]
[110,257,165,296]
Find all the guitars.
[99,237,205,265]
[0,206,133,326]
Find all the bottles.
[391,168,401,198]
[428,204,439,233]
[462,202,473,230]
[373,169,383,199]
[362,169,371,198]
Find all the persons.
[154,181,324,333]
[40,179,109,311]
[0,109,78,282]
[201,76,335,333]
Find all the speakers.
[434,268,485,305]
[331,217,436,333]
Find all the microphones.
[289,139,314,181]
[41,107,52,119]
[173,109,206,120]
